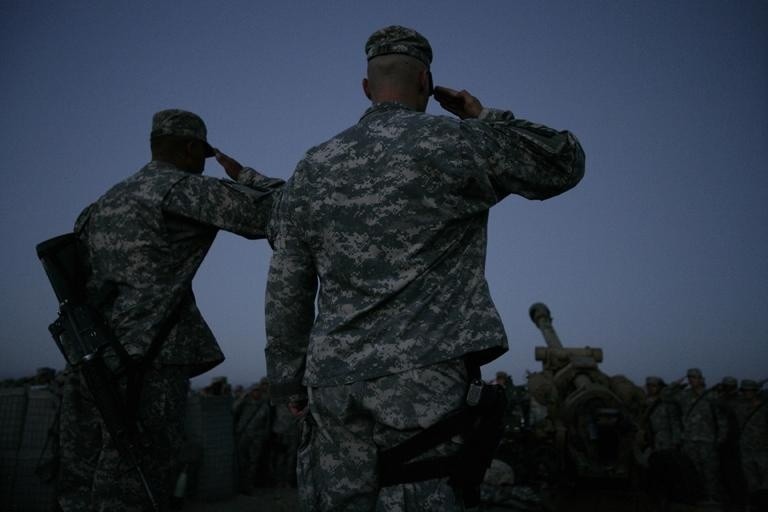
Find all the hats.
[365,26,432,66]
[646,367,759,390]
[496,372,508,379]
[210,376,267,392]
[34,368,56,378]
[152,109,216,158]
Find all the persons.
[264,25,585,512]
[50,108,287,512]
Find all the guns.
[35,232,171,512]
